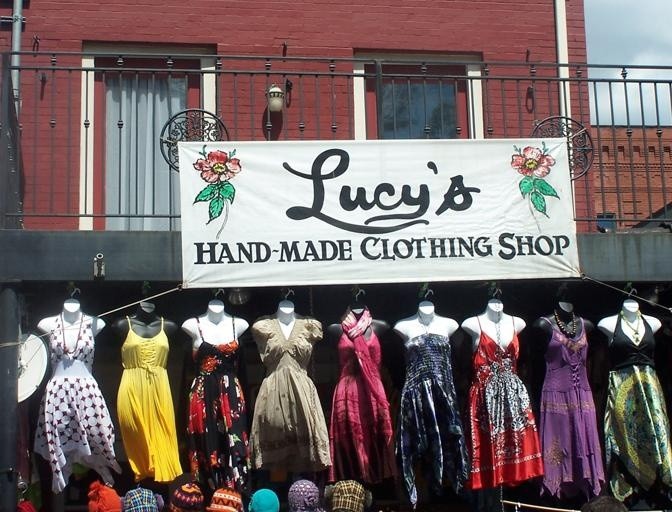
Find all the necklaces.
[417,309,436,336]
[552,308,577,338]
[617,308,642,342]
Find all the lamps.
[265,83,284,113]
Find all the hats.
[247,489,280,512]
[324,479,372,512]
[168,483,204,512]
[88,482,122,512]
[288,479,323,512]
[206,487,243,512]
[123,488,165,512]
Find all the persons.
[249,298,332,473]
[460,296,544,492]
[34,294,122,495]
[532,300,608,504]
[392,299,469,509]
[107,301,181,484]
[324,301,399,484]
[594,298,671,502]
[180,298,252,492]
